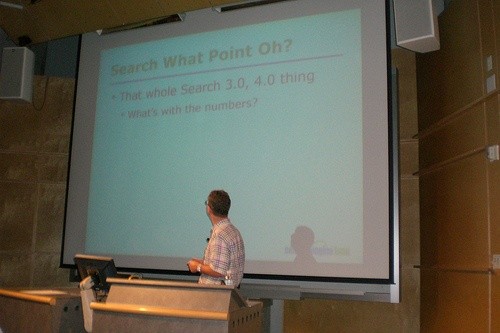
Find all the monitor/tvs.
[74,254,118,289]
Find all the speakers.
[0,47,35,103]
[393,0,441,53]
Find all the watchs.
[197,263,203,273]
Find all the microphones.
[206,238,209,242]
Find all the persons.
[188,190,245,288]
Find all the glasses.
[205,200,212,210]
